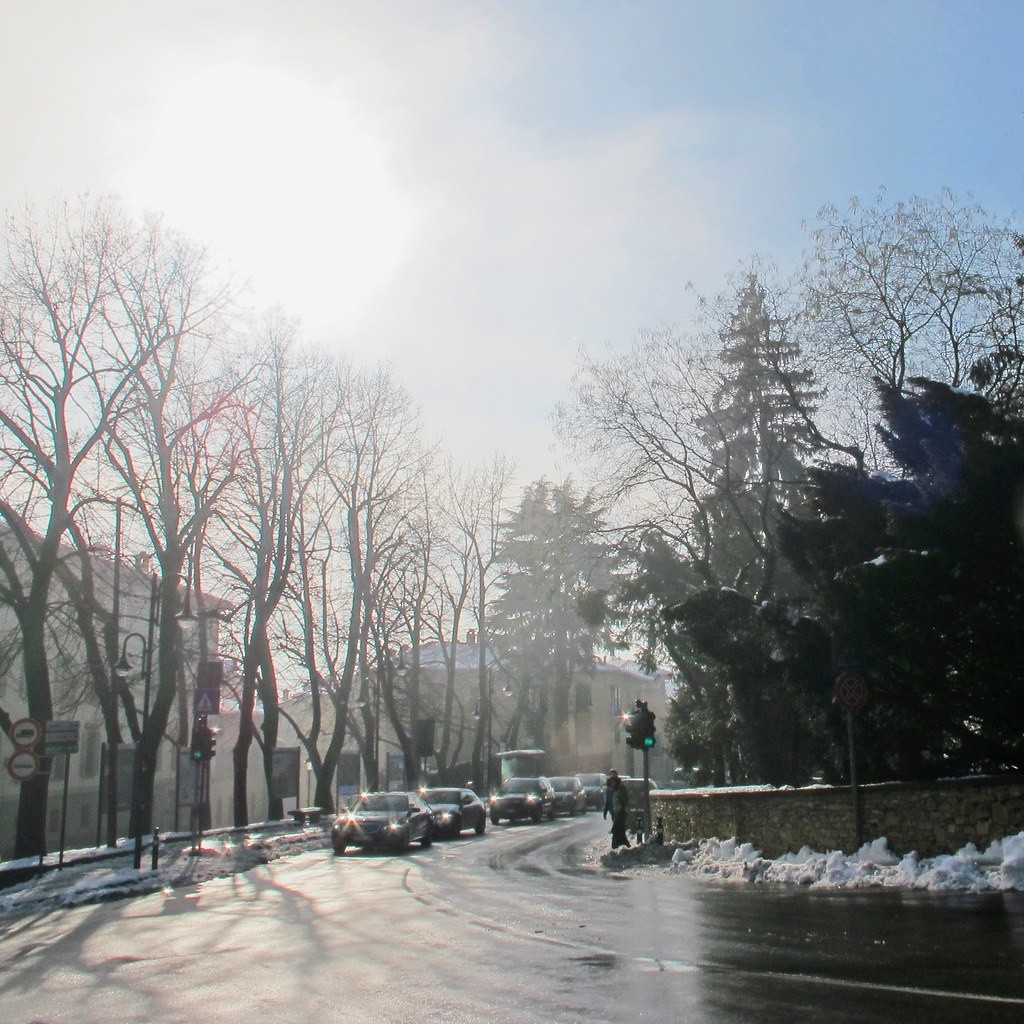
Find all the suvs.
[487,776,557,823]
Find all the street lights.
[373,640,409,791]
[113,630,156,868]
[132,573,201,871]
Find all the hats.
[607,776,621,787]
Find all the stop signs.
[8,750,40,783]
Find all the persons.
[603,769,631,849]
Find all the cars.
[332,791,437,851]
[671,767,699,792]
[411,787,487,840]
[575,771,609,811]
[546,776,588,817]
[621,778,657,818]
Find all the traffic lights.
[206,727,216,761]
[191,724,206,762]
[624,714,638,750]
[639,711,656,750]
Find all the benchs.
[287,807,323,823]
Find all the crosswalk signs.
[193,688,219,716]
